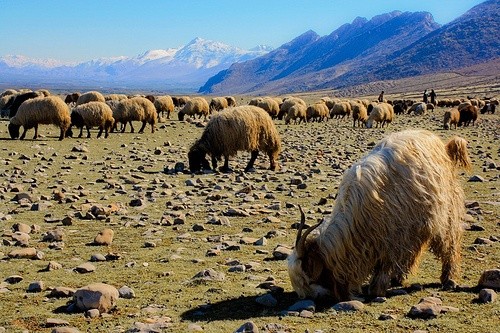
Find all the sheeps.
[0,88,238,141]
[287,128,471,309]
[188,105,282,173]
[248,95,500,131]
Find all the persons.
[378,91,384,102]
[467,96,473,100]
[429,89,436,109]
[423,89,429,104]
